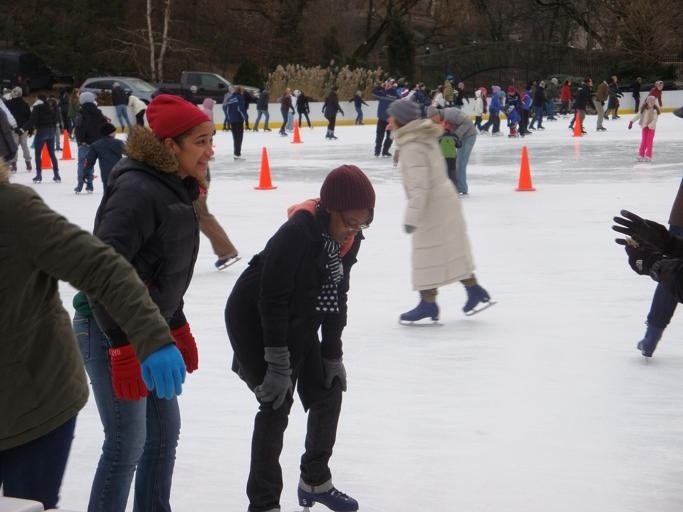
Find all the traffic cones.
[572,112,584,139]
[59,129,73,162]
[291,121,304,145]
[40,145,54,171]
[253,146,276,192]
[513,146,538,193]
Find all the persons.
[224,165,375,512]
[184,84,242,271]
[0,157,186,512]
[424,105,477,196]
[0,75,148,193]
[223,85,370,160]
[371,75,665,162]
[637,106,682,357]
[72,94,214,512]
[386,99,497,326]
[611,210,682,304]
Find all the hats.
[491,85,501,93]
[507,86,515,92]
[10,87,22,97]
[112,82,120,88]
[447,75,455,80]
[552,78,558,81]
[320,165,377,211]
[480,87,487,93]
[146,95,212,140]
[78,92,97,105]
[655,81,664,85]
[499,91,506,97]
[645,95,656,103]
[387,98,421,125]
[427,106,439,117]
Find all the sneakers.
[399,299,443,328]
[537,125,545,129]
[329,135,338,140]
[263,127,272,132]
[462,283,497,318]
[529,125,536,130]
[635,157,643,162]
[297,478,358,512]
[233,155,246,161]
[598,126,607,131]
[457,188,468,195]
[85,187,93,194]
[492,130,503,136]
[642,157,651,162]
[481,125,490,136]
[52,176,61,182]
[278,131,289,138]
[73,187,81,194]
[382,152,393,157]
[375,153,380,157]
[32,176,41,184]
[326,135,330,139]
[636,337,658,365]
[252,127,259,132]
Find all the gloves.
[404,225,417,234]
[108,342,149,401]
[625,235,664,277]
[168,324,198,374]
[323,358,348,392]
[610,209,669,248]
[140,344,187,401]
[628,121,633,129]
[253,365,293,411]
[82,174,89,183]
